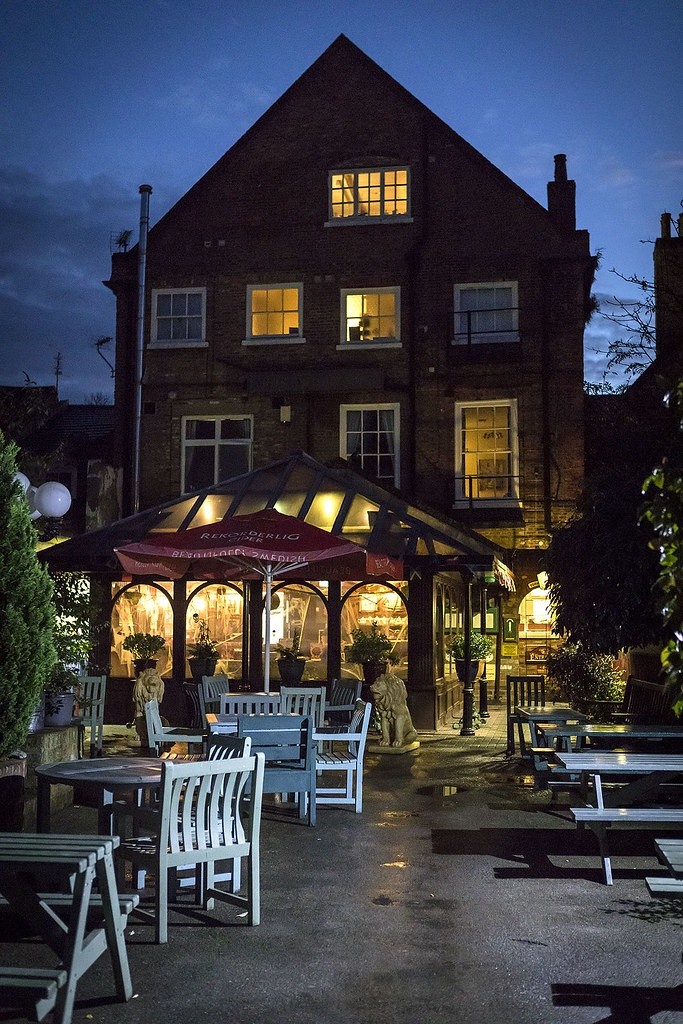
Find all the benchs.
[581,674,672,751]
[0,966,68,1000]
[531,747,683,808]
[0,892,140,915]
[645,877,683,899]
[569,808,683,886]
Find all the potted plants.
[444,630,496,683]
[186,619,222,679]
[344,621,401,685]
[273,628,305,684]
[121,633,166,678]
[41,568,111,726]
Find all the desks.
[205,712,300,803]
[653,838,683,881]
[35,758,200,893]
[514,706,587,771]
[539,725,683,753]
[0,831,132,1024]
[553,752,683,808]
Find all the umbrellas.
[115,505,403,696]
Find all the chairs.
[74,675,106,759]
[507,674,549,756]
[118,672,371,944]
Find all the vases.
[27,689,45,734]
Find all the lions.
[369,673,418,749]
[132,668,165,718]
